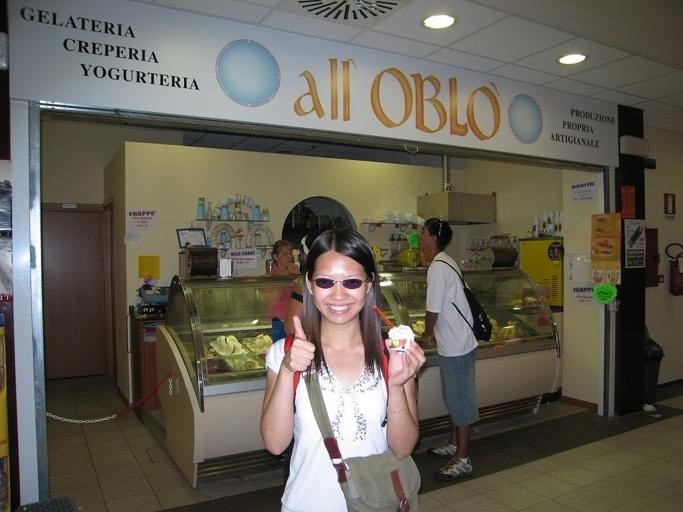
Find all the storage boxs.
[415,190,496,224]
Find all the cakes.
[388,323,416,351]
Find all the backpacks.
[435,257,493,341]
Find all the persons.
[261,239,301,322]
[258,225,427,512]
[410,215,481,487]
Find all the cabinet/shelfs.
[134,320,160,413]
[190,218,275,251]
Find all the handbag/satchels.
[302,370,421,512]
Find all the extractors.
[416,154,496,226]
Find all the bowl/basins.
[359,216,406,223]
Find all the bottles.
[220,226,261,250]
[195,194,268,220]
[533,207,562,236]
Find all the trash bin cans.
[643,338,664,404]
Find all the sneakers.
[434,454,473,483]
[429,443,457,458]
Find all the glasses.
[310,275,368,289]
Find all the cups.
[443,183,455,193]
[386,333,413,352]
[470,238,491,249]
